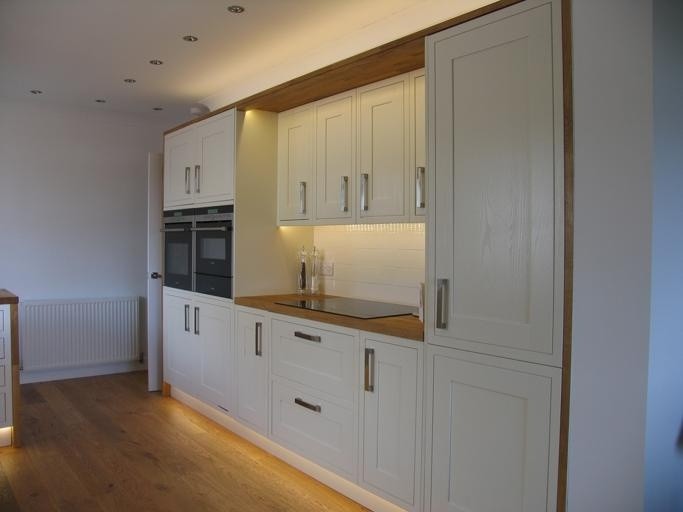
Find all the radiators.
[19,295,143,368]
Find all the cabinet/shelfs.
[273,100,314,227]
[424,342,572,512]
[0,288,23,452]
[161,284,231,427]
[423,0,571,365]
[269,310,360,484]
[313,72,409,226]
[159,107,314,212]
[410,65,427,222]
[361,315,425,510]
[232,304,269,444]
